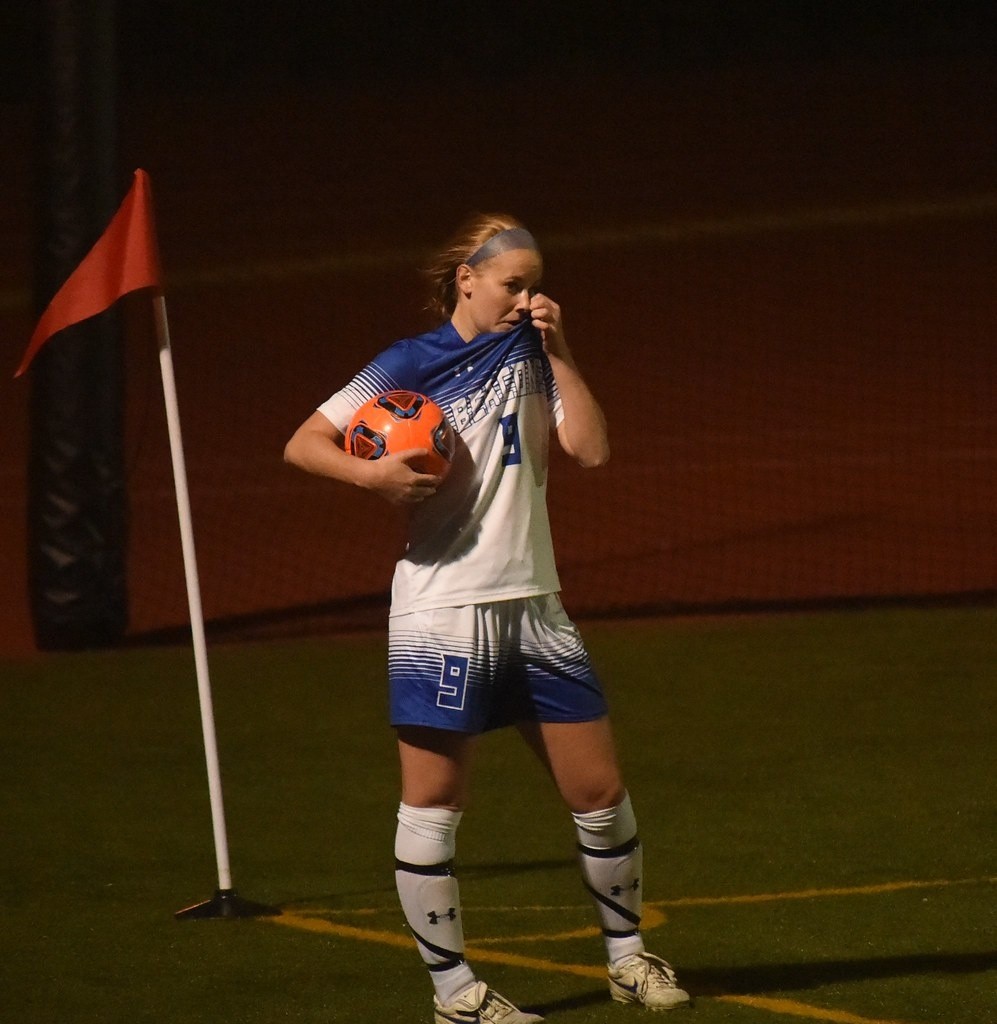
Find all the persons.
[282,210,695,1024]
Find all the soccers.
[345,392,457,495]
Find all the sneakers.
[431,984,543,1024]
[607,954,689,1010]
